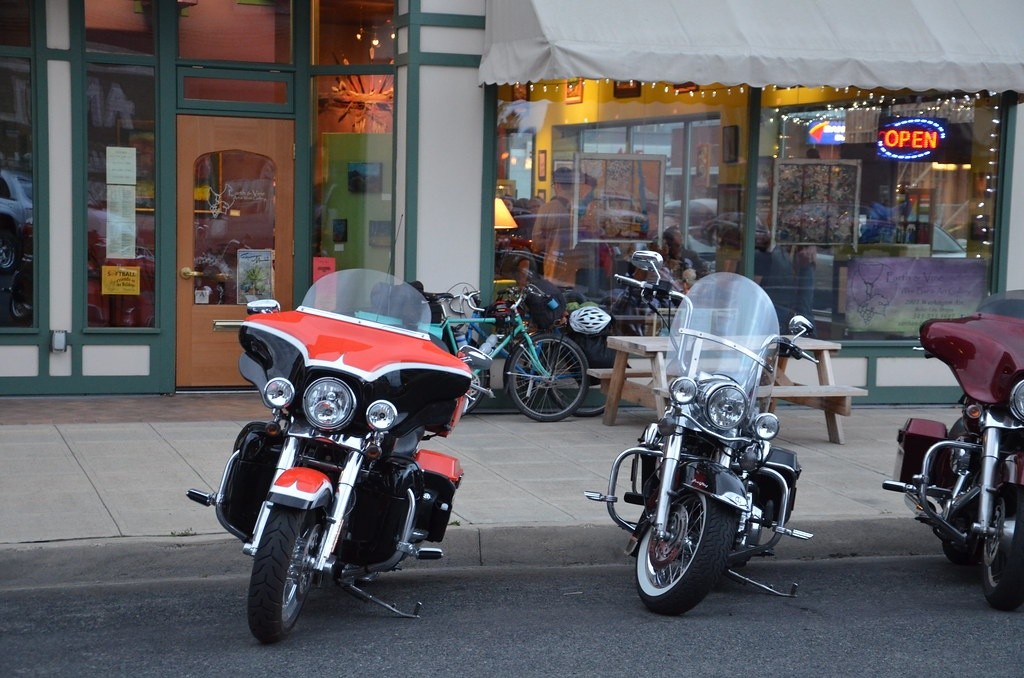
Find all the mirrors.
[506,126,536,199]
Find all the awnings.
[478,0,1024,104]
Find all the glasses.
[551,183,555,189]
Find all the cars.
[0,170,38,277]
[491,194,840,318]
[932,223,967,259]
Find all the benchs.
[757,385,868,397]
[587,369,652,379]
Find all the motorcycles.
[880,288,1022,614]
[187,269,497,644]
[583,251,822,614]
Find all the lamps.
[494,197,518,229]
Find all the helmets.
[570,304,613,334]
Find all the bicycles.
[357,282,630,424]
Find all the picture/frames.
[511,81,529,116]
[613,79,641,98]
[722,124,739,163]
[538,189,546,203]
[565,78,583,105]
[538,150,547,181]
[717,184,742,250]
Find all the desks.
[600,335,852,444]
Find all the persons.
[599,226,709,281]
[499,167,600,288]
[754,219,817,340]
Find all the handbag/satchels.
[566,322,631,388]
[526,280,565,328]
